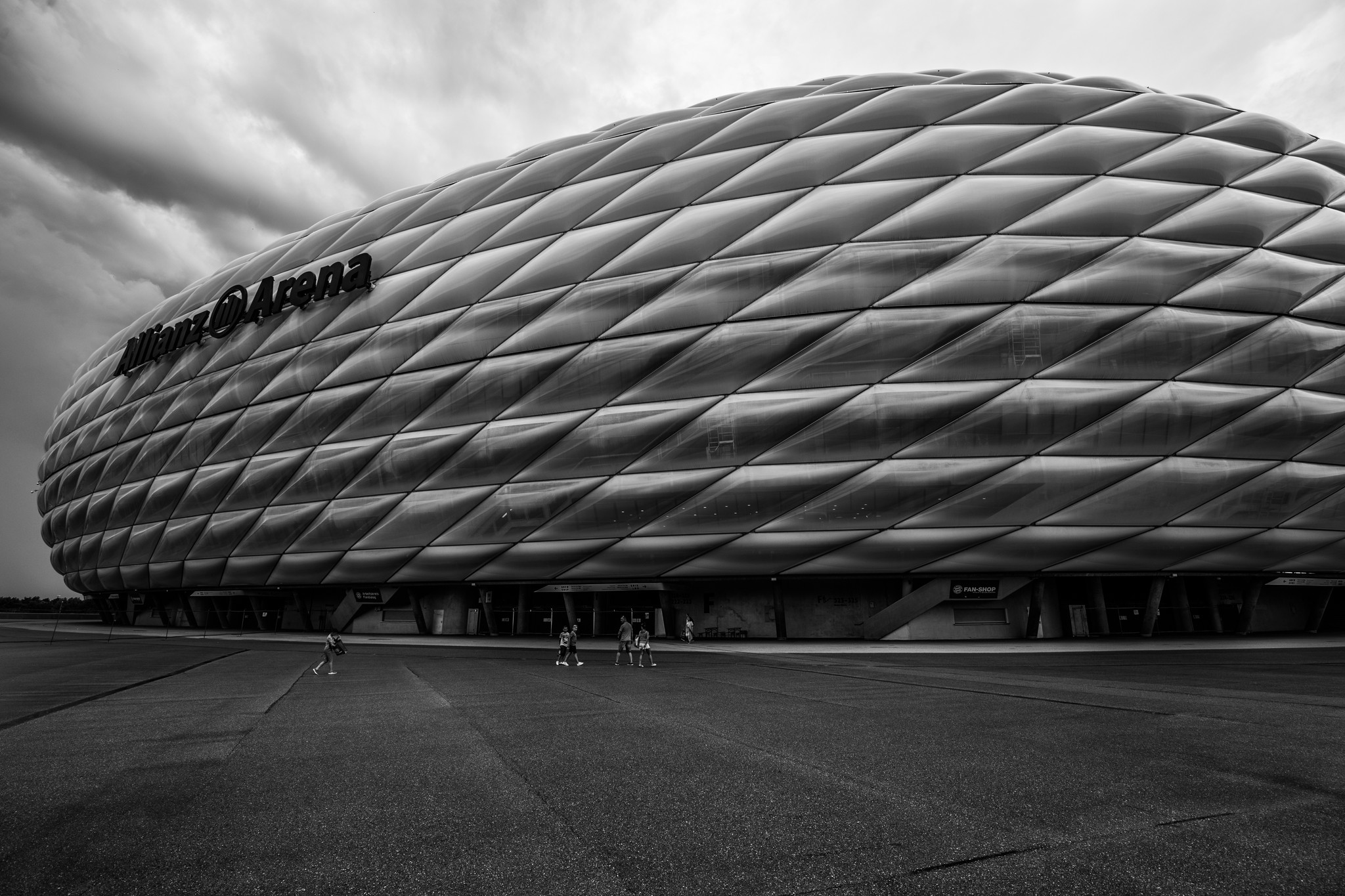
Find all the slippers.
[651,664,657,667]
[638,665,644,668]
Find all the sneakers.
[328,670,337,674]
[627,662,633,665]
[577,662,583,666]
[556,661,559,665]
[559,661,563,664]
[312,668,319,675]
[562,662,569,666]
[615,662,619,665]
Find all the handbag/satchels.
[680,630,686,641]
[556,648,561,657]
[633,635,640,649]
[331,636,348,656]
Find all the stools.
[739,630,747,638]
[735,633,740,638]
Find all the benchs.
[698,632,710,637]
[713,632,726,637]
[721,632,734,638]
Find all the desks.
[704,627,718,638]
[726,627,742,638]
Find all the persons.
[639,623,656,667]
[615,616,633,665]
[313,628,340,674]
[556,624,583,666]
[683,614,694,644]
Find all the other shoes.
[687,641,690,644]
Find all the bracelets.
[691,629,692,630]
